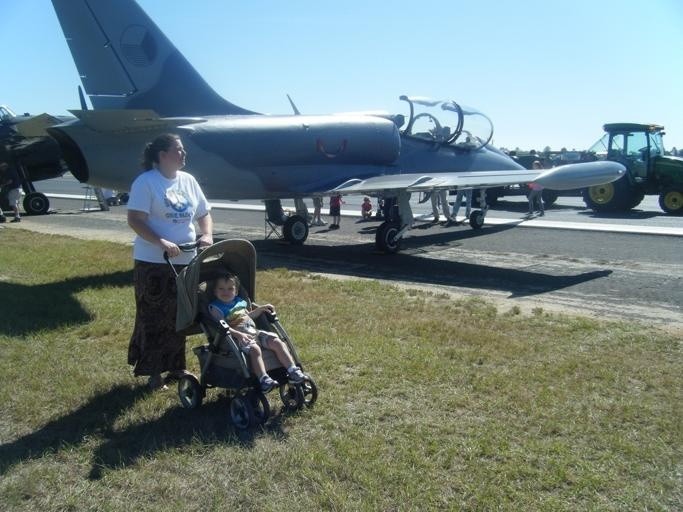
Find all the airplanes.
[0,86,87,216]
[11,0,627,253]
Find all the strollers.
[164,237,319,430]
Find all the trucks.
[449,123,683,216]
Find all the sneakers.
[168,370,197,383]
[286,368,310,383]
[148,377,168,394]
[259,375,279,393]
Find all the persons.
[430,188,456,225]
[328,195,346,230]
[0,162,23,222]
[209,270,311,392]
[293,197,313,224]
[526,160,546,218]
[450,189,471,221]
[125,131,214,391]
[361,196,372,219]
[309,195,326,226]
